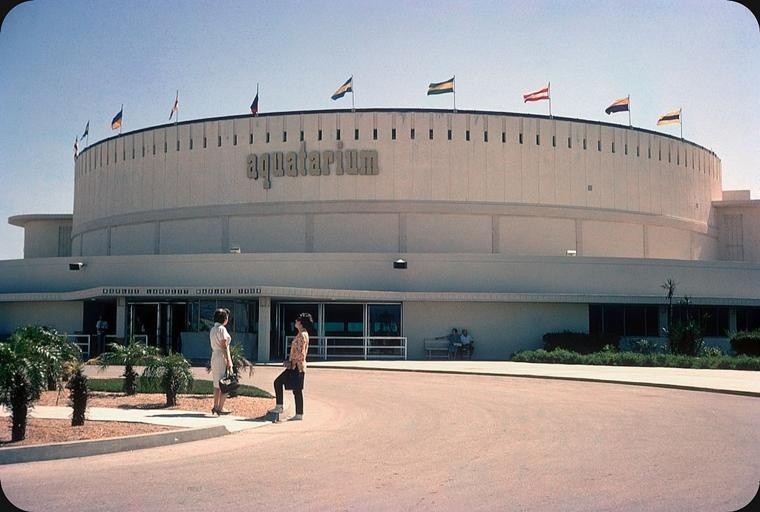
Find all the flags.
[605,97,629,115]
[169,95,178,120]
[81,122,89,141]
[523,85,550,103]
[331,77,353,100]
[73,139,77,160]
[657,109,680,127]
[250,93,258,117]
[427,78,453,95]
[111,110,122,130]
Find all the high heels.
[217,408,231,415]
[212,408,219,414]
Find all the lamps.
[392,259,408,270]
[69,262,88,272]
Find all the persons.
[267,313,313,420]
[210,308,233,416]
[460,329,474,360]
[95,315,108,355]
[135,317,146,334]
[435,328,460,361]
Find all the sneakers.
[267,404,283,413]
[287,414,302,420]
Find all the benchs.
[425,337,470,360]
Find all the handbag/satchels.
[219,375,240,393]
[285,372,304,390]
